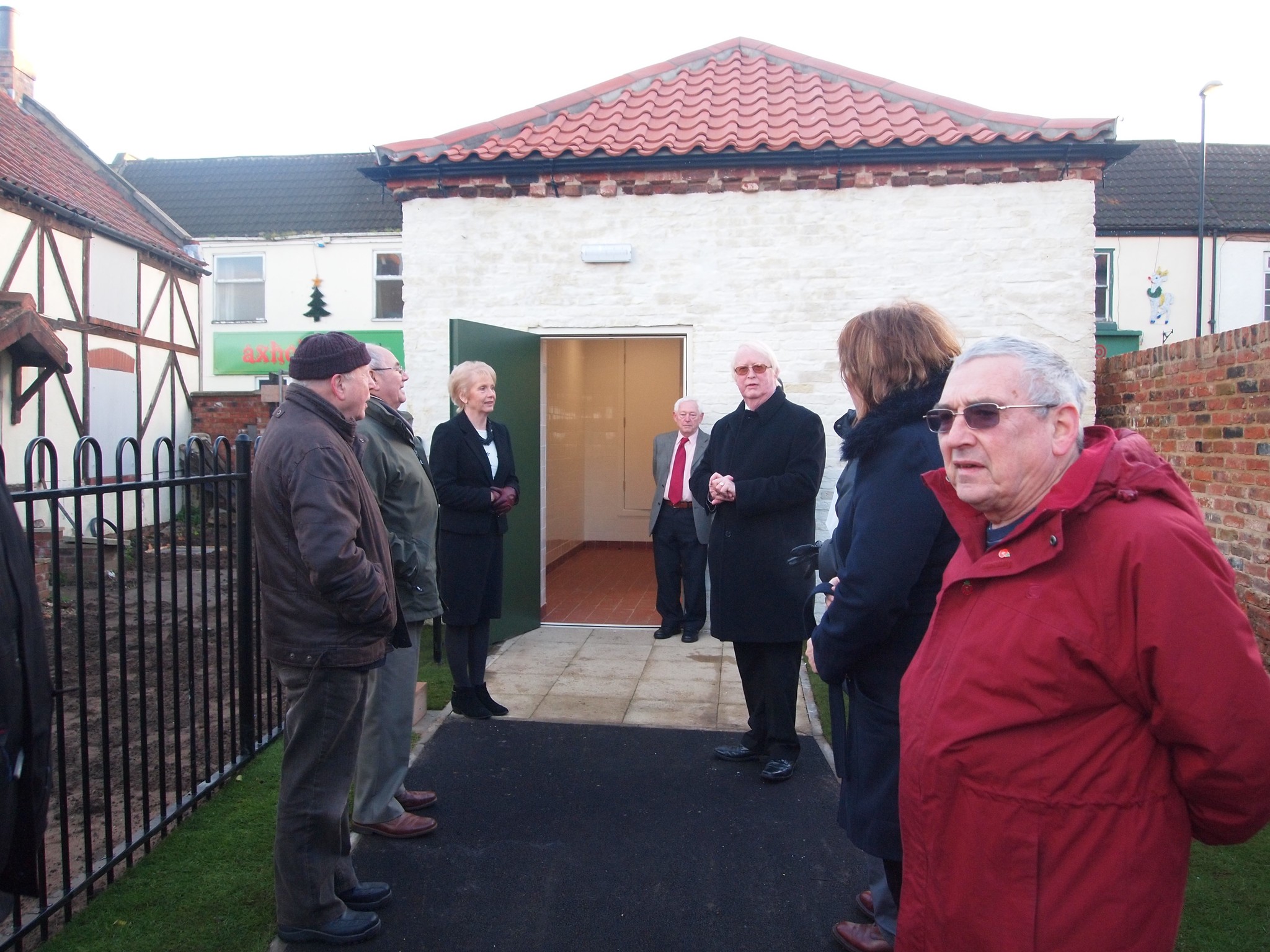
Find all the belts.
[662,499,693,509]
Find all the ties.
[668,437,690,504]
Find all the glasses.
[734,364,772,375]
[923,402,1056,433]
[370,366,402,371]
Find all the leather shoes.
[855,890,875,920]
[351,811,437,838]
[336,881,392,911]
[396,790,438,812]
[714,742,770,761]
[761,759,798,781]
[276,905,381,944]
[654,626,681,639]
[832,920,894,952]
[681,627,699,642]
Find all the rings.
[719,482,724,487]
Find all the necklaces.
[472,417,494,445]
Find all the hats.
[288,331,372,380]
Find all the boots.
[474,682,509,716]
[451,685,493,719]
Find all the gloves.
[787,540,823,582]
[490,486,517,517]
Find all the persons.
[429,358,523,722]
[0,444,60,903]
[249,332,418,947]
[647,396,715,643]
[350,341,451,841]
[688,342,829,783]
[892,332,1270,951]
[804,300,968,915]
[814,397,903,951]
